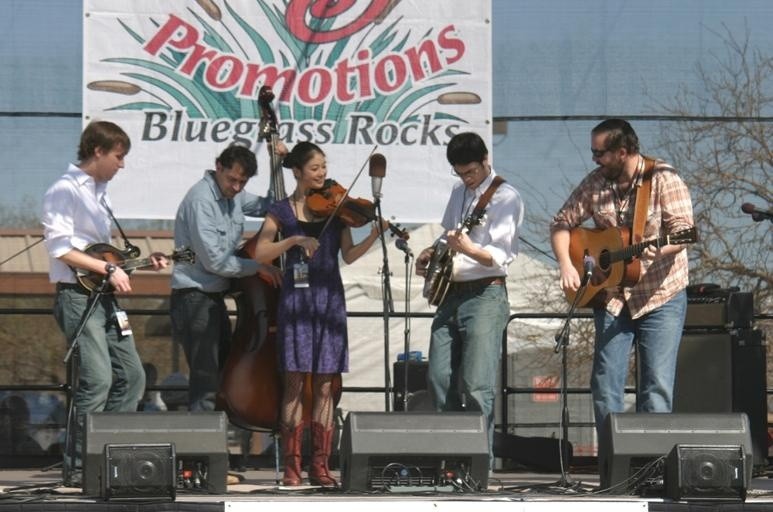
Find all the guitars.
[560,225,698,310]
[422,208,487,307]
[74,241,196,295]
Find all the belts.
[449,276,506,290]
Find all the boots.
[308,420,338,487]
[279,419,305,486]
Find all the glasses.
[450,159,482,177]
[590,147,610,157]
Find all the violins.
[306,178,409,244]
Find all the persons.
[256,143,389,487]
[550,120,694,495]
[416,133,524,486]
[170,140,289,484]
[42,122,172,487]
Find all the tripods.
[496,277,615,497]
[40,278,114,496]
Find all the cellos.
[219,85,344,435]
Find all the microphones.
[395,239,414,257]
[369,153,386,201]
[583,256,595,277]
[104,262,116,276]
[741,203,772,216]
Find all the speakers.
[100,444,177,502]
[665,444,747,502]
